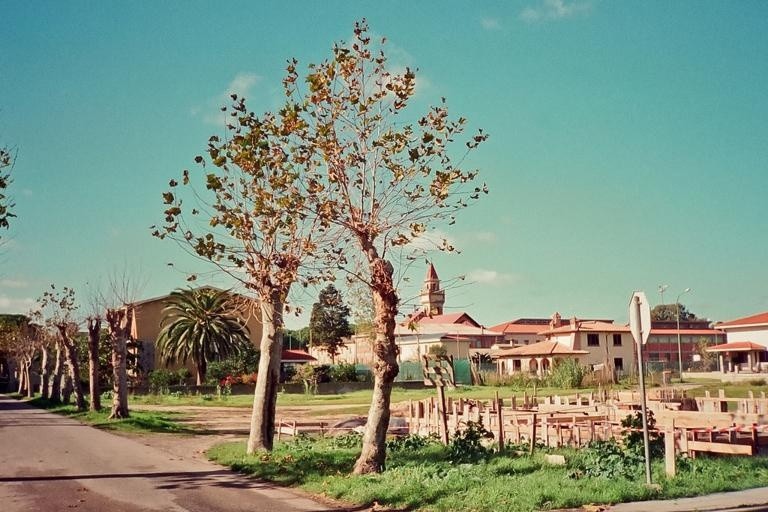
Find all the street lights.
[675,288,689,376]
